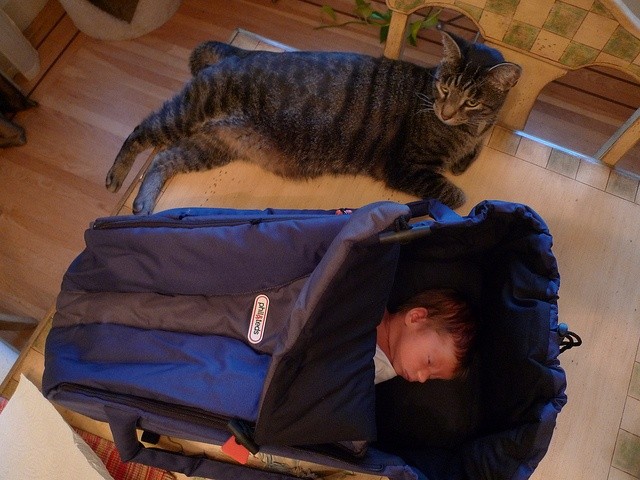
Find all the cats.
[104,27,523,219]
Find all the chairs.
[384,0,639,166]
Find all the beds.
[41,198,582,479]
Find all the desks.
[0,27,640,480]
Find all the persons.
[372,288,478,385]
